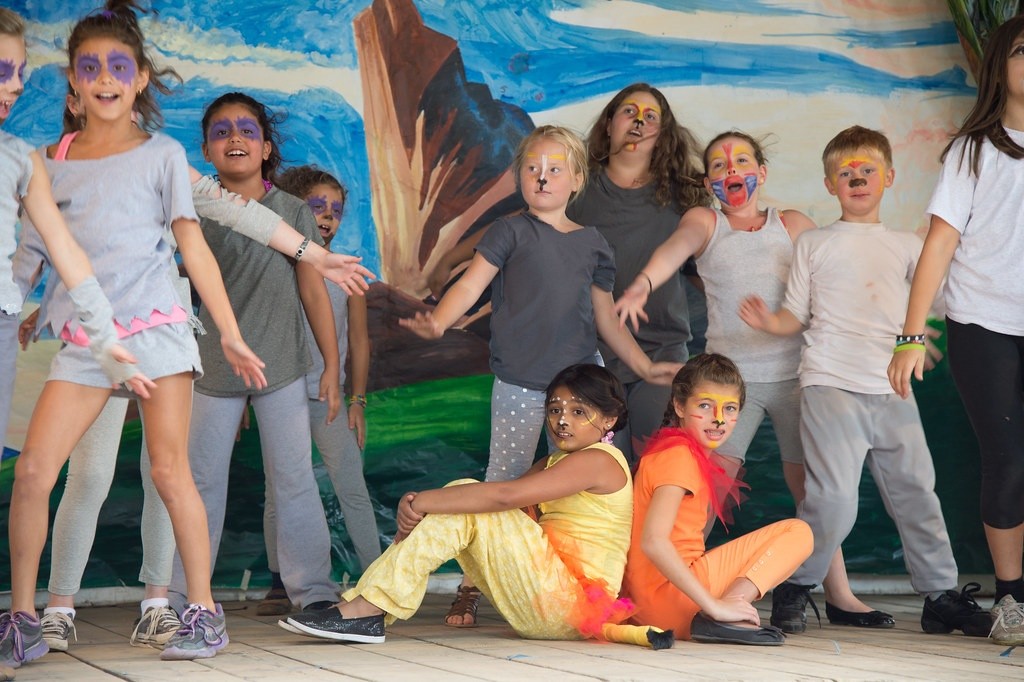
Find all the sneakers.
[159,603,230,659]
[768,581,821,634]
[0,611,49,668]
[987,594,1024,645]
[921,582,992,636]
[278,619,327,639]
[287,607,388,643]
[130,605,184,647]
[40,611,78,650]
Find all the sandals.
[444,584,483,627]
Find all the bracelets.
[637,271,652,296]
[294,237,311,261]
[346,395,367,410]
[894,333,926,352]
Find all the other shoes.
[691,611,787,645]
[256,589,290,614]
[303,601,338,612]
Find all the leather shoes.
[825,602,895,628]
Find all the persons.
[738,126,995,638]
[256,162,382,616]
[612,125,895,630]
[166,94,344,620]
[565,82,716,478]
[0,6,160,669]
[887,17,1024,648]
[0,0,270,667]
[620,351,815,647]
[399,123,686,629]
[277,363,634,640]
